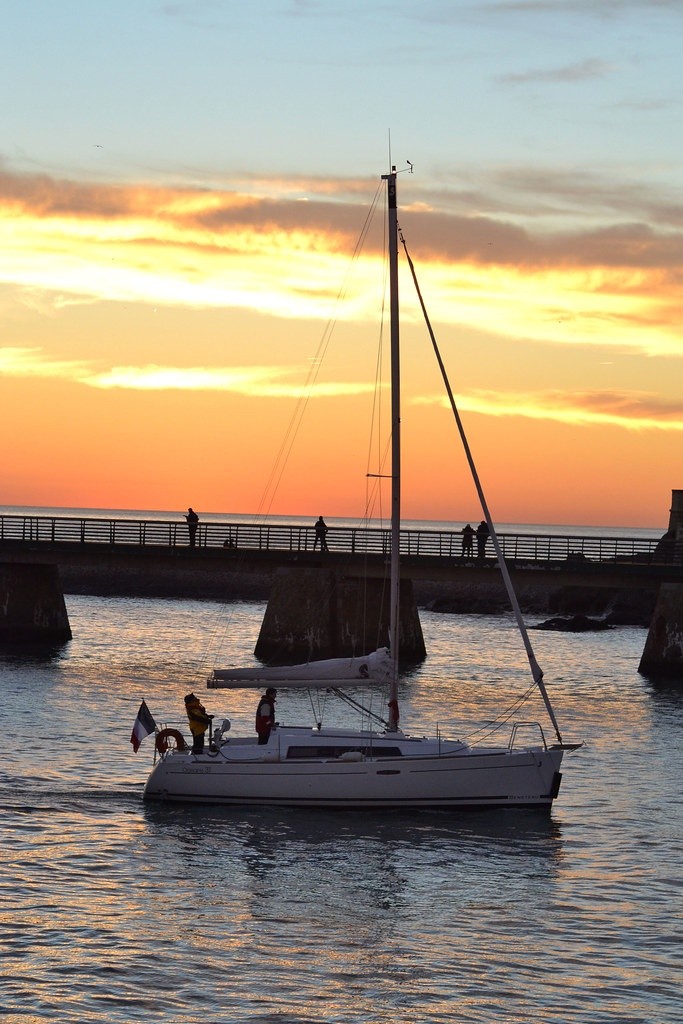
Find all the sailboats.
[127,128,584,813]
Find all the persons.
[185,508,199,546]
[460,521,490,558]
[184,692,212,755]
[256,688,277,745]
[313,516,329,551]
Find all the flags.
[130,700,156,754]
